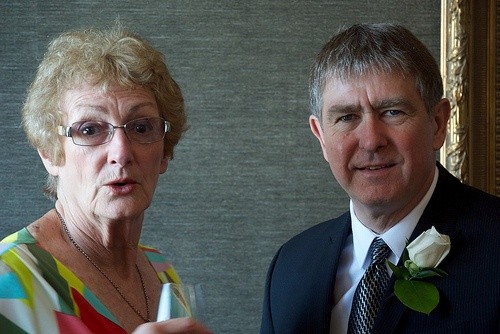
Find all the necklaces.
[55,209,151,323]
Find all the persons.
[0,28,212,334]
[259,22,499,334]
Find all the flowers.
[385,225,451,316]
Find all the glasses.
[58,118,170,146]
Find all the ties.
[347,237,393,334]
